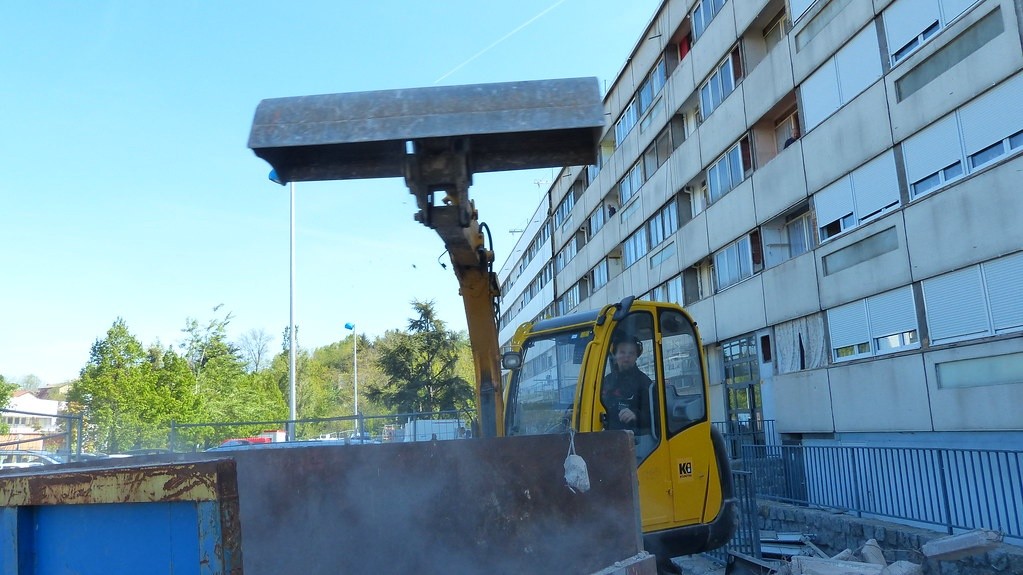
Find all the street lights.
[346,322,360,442]
[266,164,302,443]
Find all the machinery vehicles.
[247,79,733,573]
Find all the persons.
[465,430,471,438]
[783,128,799,148]
[607,204,615,217]
[458,427,466,439]
[562,335,653,433]
[430,434,437,440]
